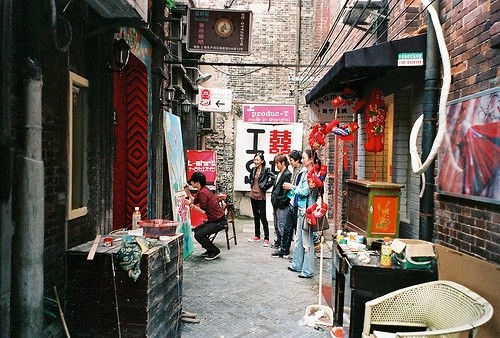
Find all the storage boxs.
[390,238,435,269]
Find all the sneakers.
[248,236,260,242]
[263,240,269,246]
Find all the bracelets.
[189,203,195,209]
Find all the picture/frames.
[437,87,500,204]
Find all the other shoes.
[270,249,289,259]
[288,266,296,271]
[271,242,283,248]
[205,249,221,260]
[298,273,312,278]
[202,251,208,256]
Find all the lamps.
[184,67,212,85]
[163,85,176,107]
[105,31,131,77]
[197,112,205,124]
[182,99,191,114]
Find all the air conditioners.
[340,0,381,23]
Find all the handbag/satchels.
[302,214,330,232]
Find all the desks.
[64,230,184,338]
[344,179,405,238]
[332,234,434,338]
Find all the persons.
[184,171,227,260]
[249,150,322,279]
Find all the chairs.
[362,280,494,338]
[202,203,237,250]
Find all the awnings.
[306,32,428,103]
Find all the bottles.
[132,207,141,230]
[380,237,392,267]
